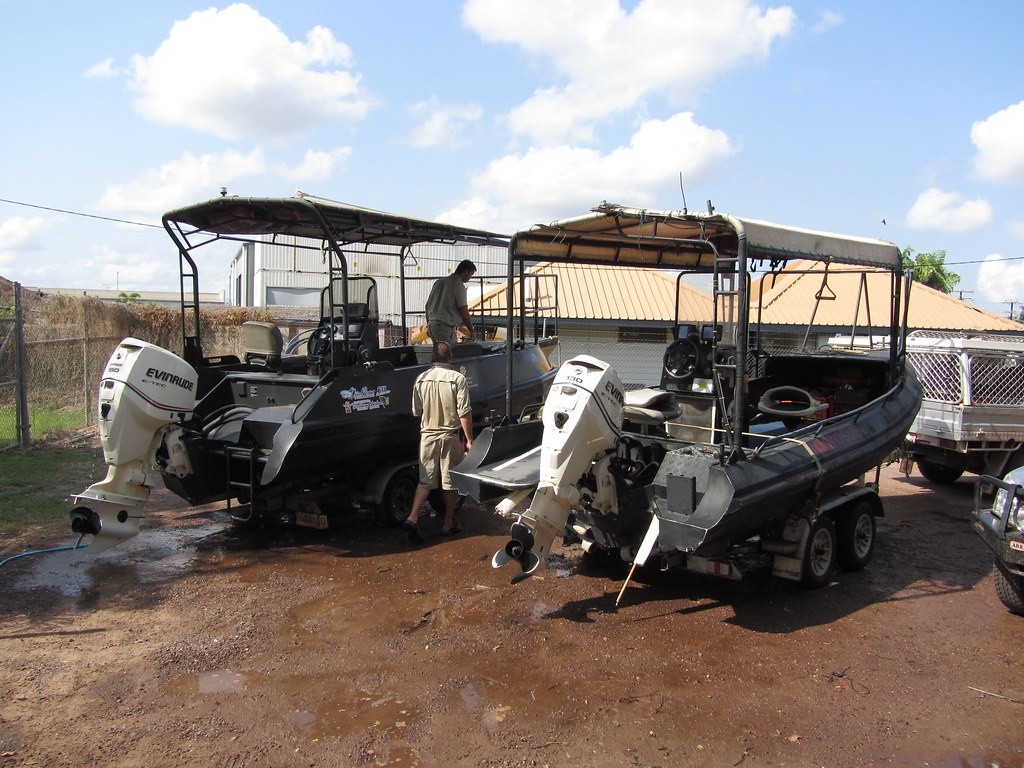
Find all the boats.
[63,189,559,563]
[447,200,926,609]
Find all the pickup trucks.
[826,329,1023,484]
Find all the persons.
[425,259,476,345]
[404,342,472,544]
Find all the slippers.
[439,518,465,535]
[404,521,424,542]
